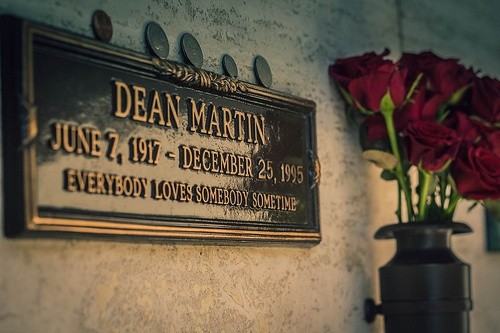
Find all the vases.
[363,220,477,332]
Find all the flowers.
[327,44,500,226]
